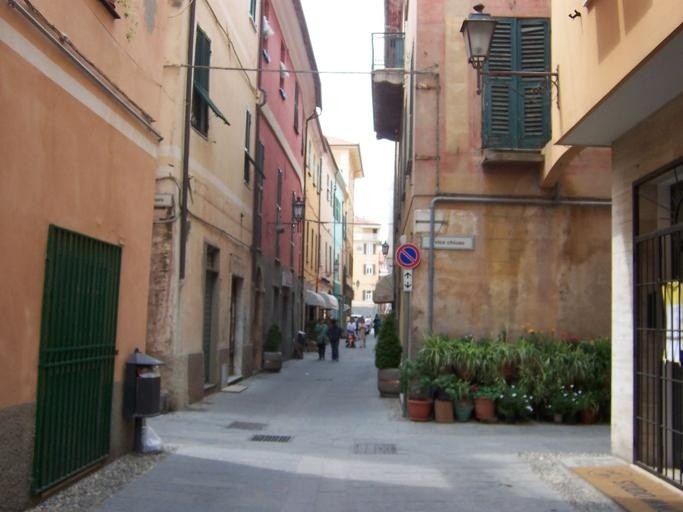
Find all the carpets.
[577,462,683,512]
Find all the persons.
[328,319,342,362]
[373,313,380,338]
[314,318,329,360]
[358,318,367,348]
[346,318,356,347]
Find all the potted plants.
[397,325,610,424]
[263,322,284,373]
[373,311,404,397]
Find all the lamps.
[457,4,562,110]
[380,241,393,262]
[267,195,306,227]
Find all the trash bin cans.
[292,330,305,358]
[125,350,162,453]
